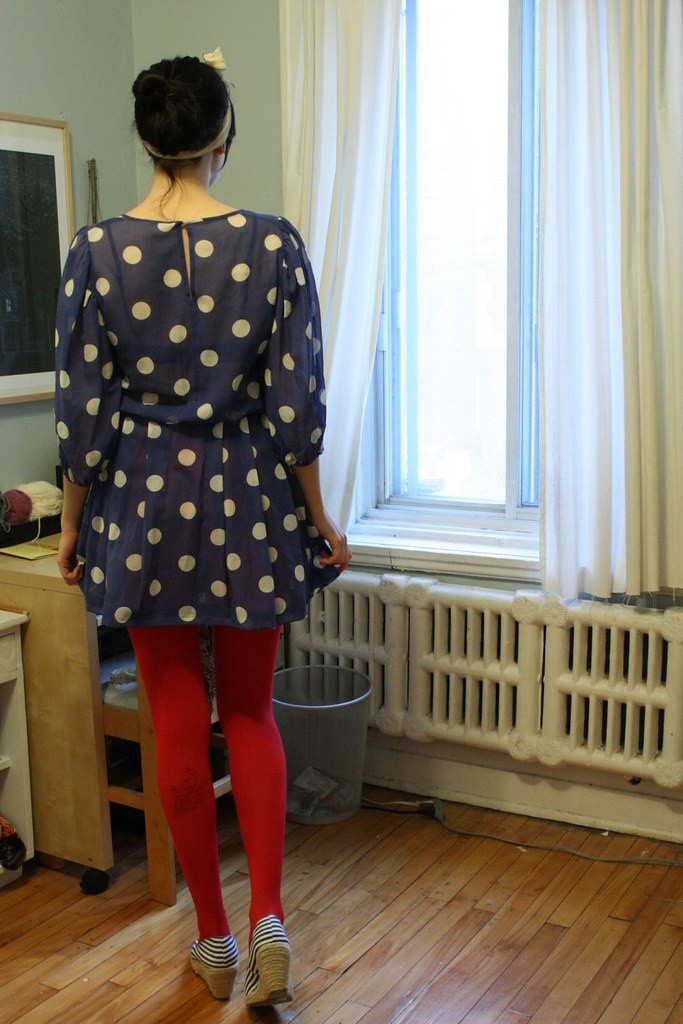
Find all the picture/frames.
[0,110,75,405]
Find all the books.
[0,543,59,562]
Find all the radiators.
[286,566,683,789]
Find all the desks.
[1,531,114,893]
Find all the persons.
[51,51,353,1013]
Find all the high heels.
[243,914,294,1007]
[190,934,239,1000]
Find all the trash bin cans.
[268,664,376,826]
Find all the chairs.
[98,621,233,908]
